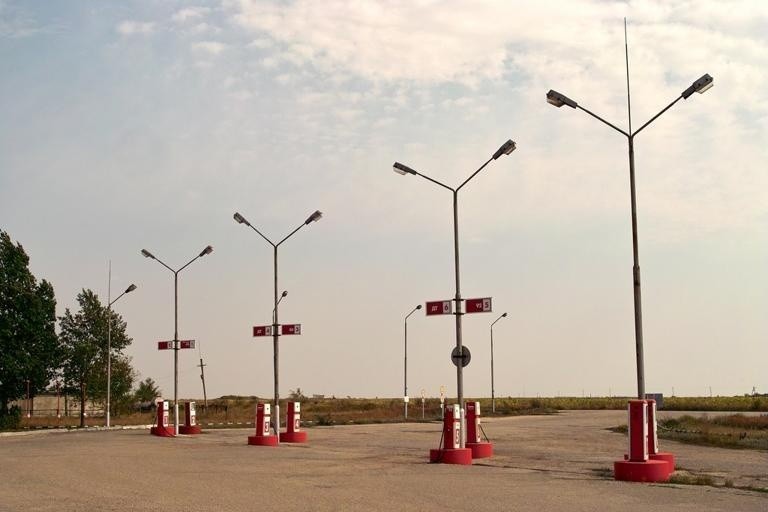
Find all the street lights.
[546,17,716,398]
[392,139,516,427]
